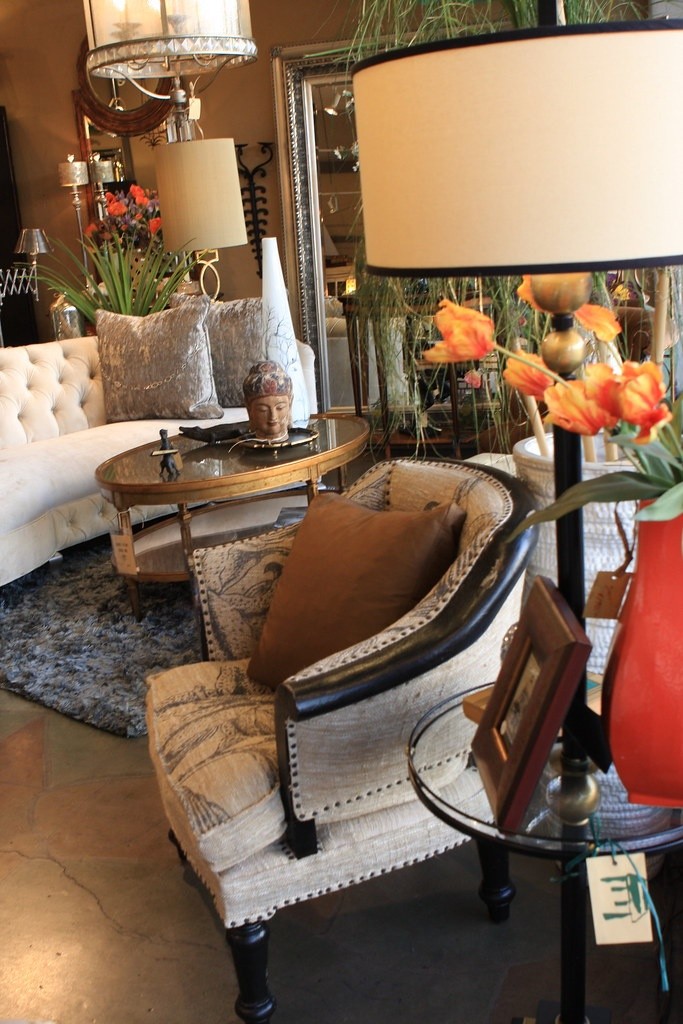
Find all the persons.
[242,362,294,443]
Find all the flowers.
[422,271,682,547]
[82,183,162,255]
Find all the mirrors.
[72,33,187,224]
[271,22,531,483]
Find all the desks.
[404,680,683,1024]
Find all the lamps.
[81,0,258,129]
[58,154,90,289]
[91,151,114,217]
[155,138,248,303]
[351,1,683,1024]
[0,228,53,307]
[259,237,311,420]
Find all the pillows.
[243,492,465,691]
[166,293,265,409]
[95,293,223,425]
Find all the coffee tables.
[95,414,371,623]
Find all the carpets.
[0,512,205,739]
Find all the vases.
[610,500,682,808]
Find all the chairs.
[147,456,539,1023]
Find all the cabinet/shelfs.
[337,290,497,459]
[0,105,41,349]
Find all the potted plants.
[303,0,683,677]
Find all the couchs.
[323,297,408,409]
[0,336,319,587]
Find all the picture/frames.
[467,577,595,836]
